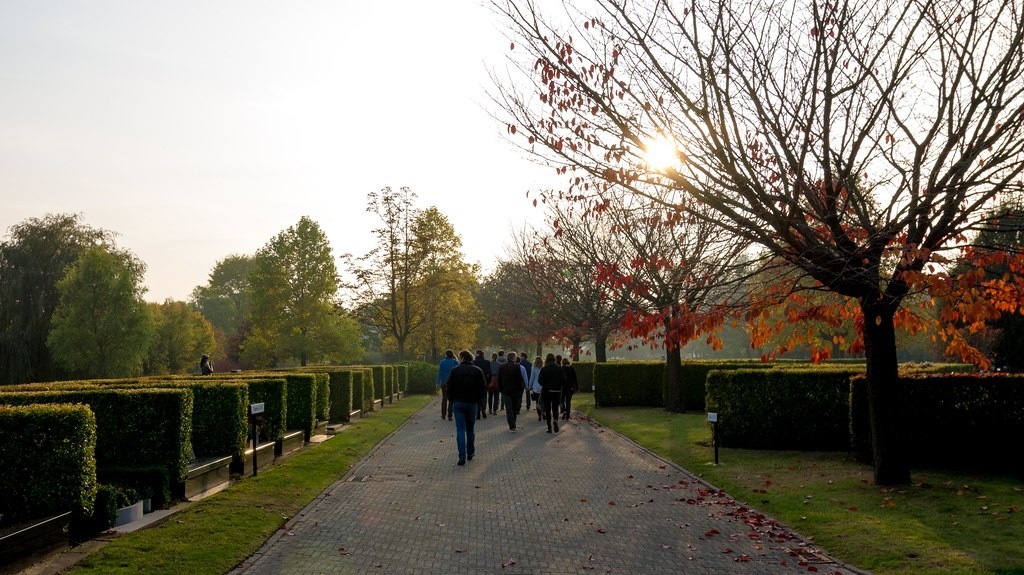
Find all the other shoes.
[543,414,546,421]
[568,416,572,419]
[467,452,474,460]
[538,418,541,421]
[510,427,515,431]
[476,417,481,419]
[493,411,497,415]
[482,411,487,419]
[553,421,559,433]
[500,408,504,411]
[489,410,492,414]
[546,429,552,433]
[562,411,567,420]
[458,460,464,465]
[449,418,453,421]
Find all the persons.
[200,354,214,375]
[434,349,578,466]
